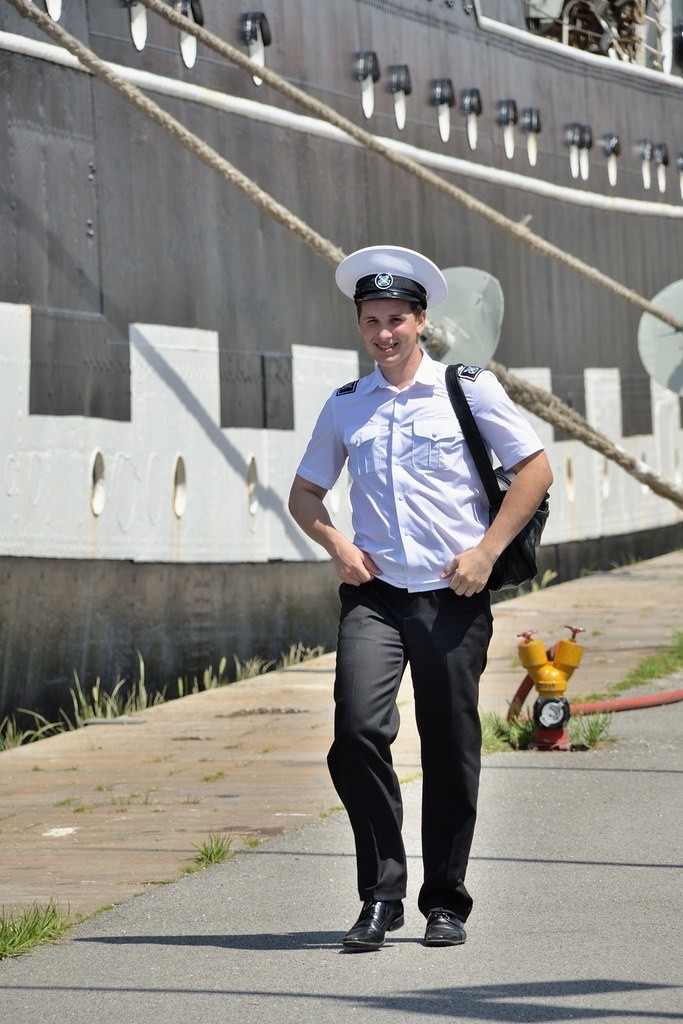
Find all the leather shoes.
[424,913,468,944]
[343,900,405,948]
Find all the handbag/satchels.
[444,363,550,593]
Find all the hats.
[335,245,449,307]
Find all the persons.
[288,244,553,949]
